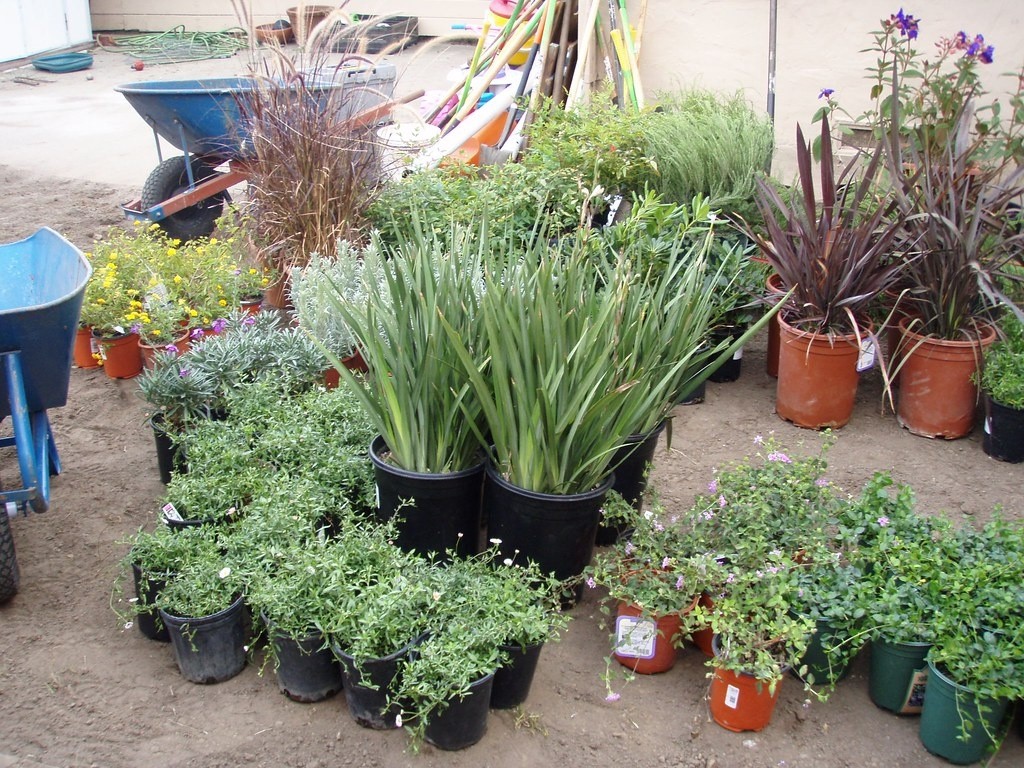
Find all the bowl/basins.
[286,5,335,45]
[256,24,292,46]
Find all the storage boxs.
[332,15,419,54]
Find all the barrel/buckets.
[272,20,291,30]
[377,123,441,183]
[489,0,540,65]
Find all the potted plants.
[300,59,1024,610]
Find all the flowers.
[75,199,569,768]
[582,423,904,726]
[810,5,992,165]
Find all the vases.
[95,326,142,380]
[785,607,848,689]
[615,569,699,675]
[287,6,335,48]
[258,603,342,702]
[489,634,547,707]
[902,162,982,202]
[153,585,249,685]
[238,295,263,316]
[144,409,219,482]
[331,631,431,730]
[76,323,97,369]
[708,633,787,731]
[129,546,173,643]
[409,651,494,750]
[137,326,191,376]
[696,595,722,658]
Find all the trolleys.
[1,225,92,607]
[107,60,425,249]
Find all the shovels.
[476,0,552,181]
